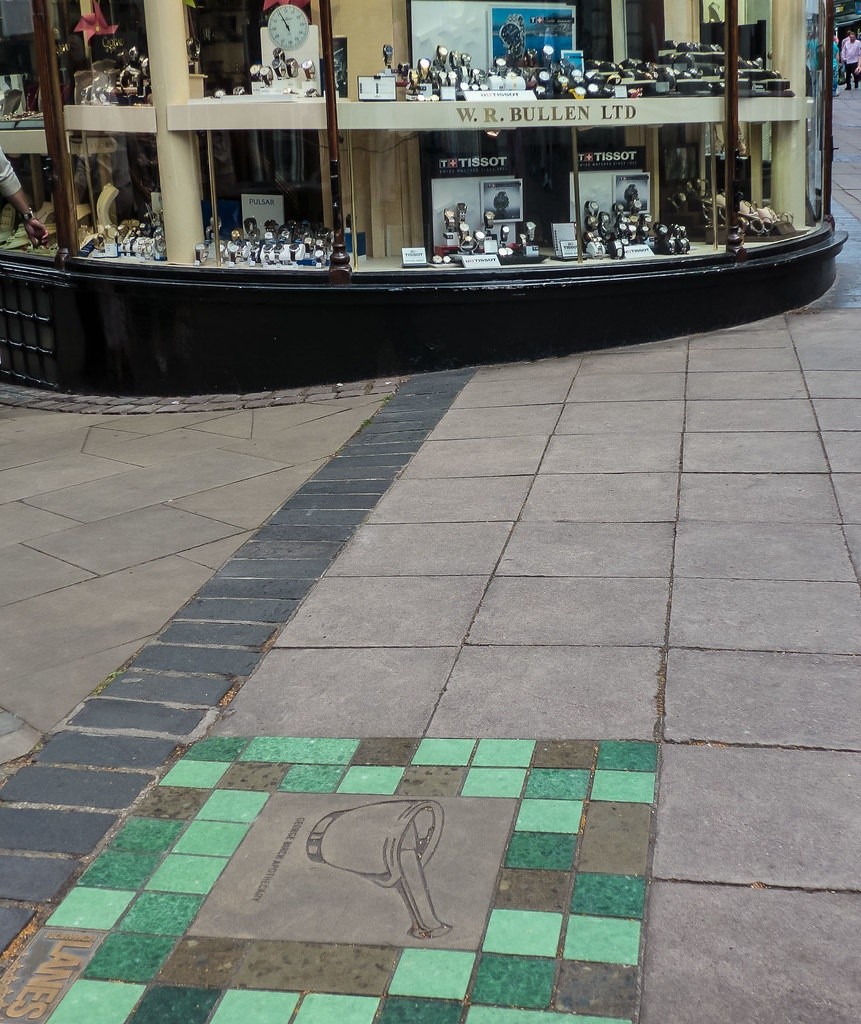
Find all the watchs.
[220,217,331,265]
[214,47,321,98]
[582,198,690,260]
[431,203,536,263]
[195,243,208,263]
[93,209,166,254]
[80,46,148,104]
[382,40,783,100]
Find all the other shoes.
[855,83,858,88]
[834,92,839,96]
[845,86,851,90]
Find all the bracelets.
[672,178,794,234]
[206,215,222,242]
[18,207,34,221]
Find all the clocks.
[268,5,309,51]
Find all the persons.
[0,148,50,247]
[841,31,861,90]
[833,36,840,97]
